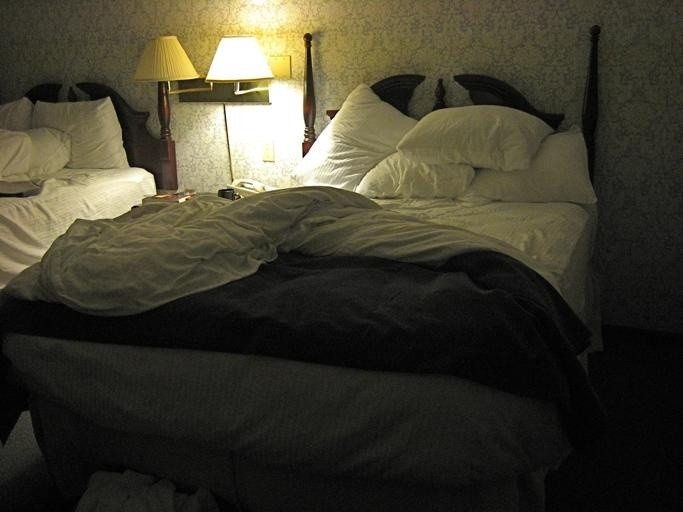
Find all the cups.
[217,188,242,201]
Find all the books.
[141,193,191,204]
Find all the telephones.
[226,179,279,198]
